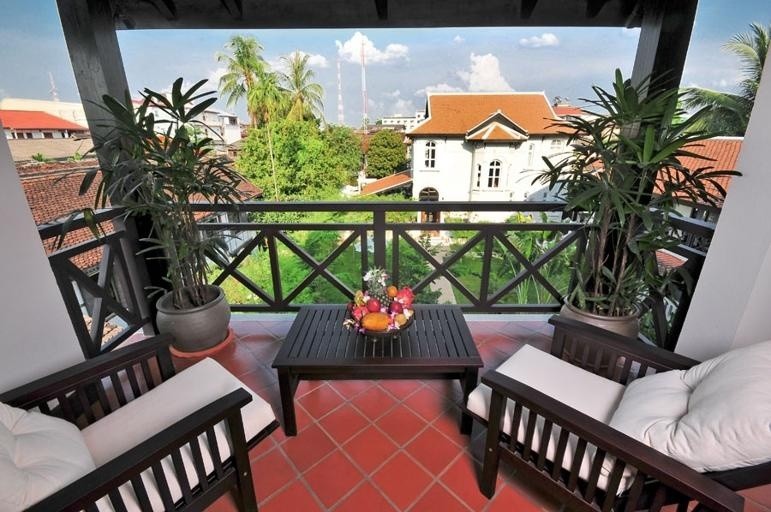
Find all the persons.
[429,212,433,222]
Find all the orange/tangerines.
[394,314,406,325]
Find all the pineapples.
[369,287,391,307]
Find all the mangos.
[362,313,389,331]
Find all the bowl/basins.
[347,313,414,342]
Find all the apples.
[367,298,381,313]
[389,302,402,314]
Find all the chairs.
[1,335,281,512]
[452,314,770,512]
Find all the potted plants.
[48,74,250,354]
[529,67,744,369]
